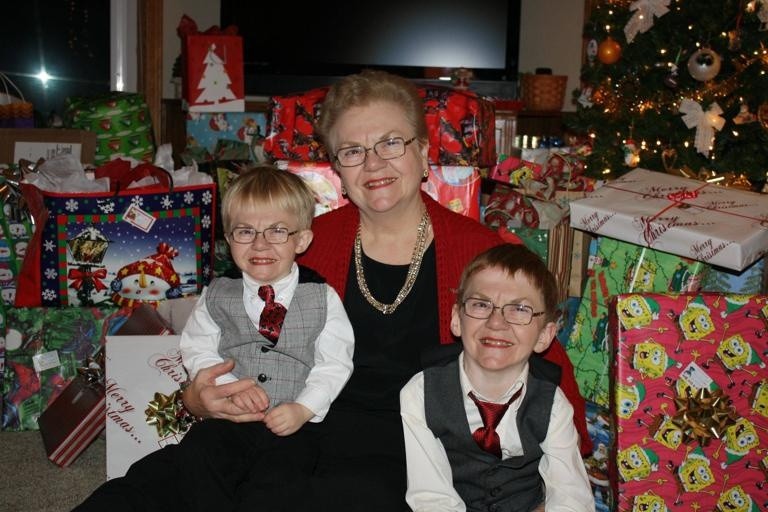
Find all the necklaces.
[354,212,430,314]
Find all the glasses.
[228,226,299,244]
[460,296,545,325]
[332,136,416,167]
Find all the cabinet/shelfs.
[244,100,580,161]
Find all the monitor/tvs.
[219,1,521,100]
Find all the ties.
[467,383,524,460]
[258,283,287,345]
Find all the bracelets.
[174,381,205,428]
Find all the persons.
[180,167,355,512]
[66,70,594,512]
[399,243,595,512]
